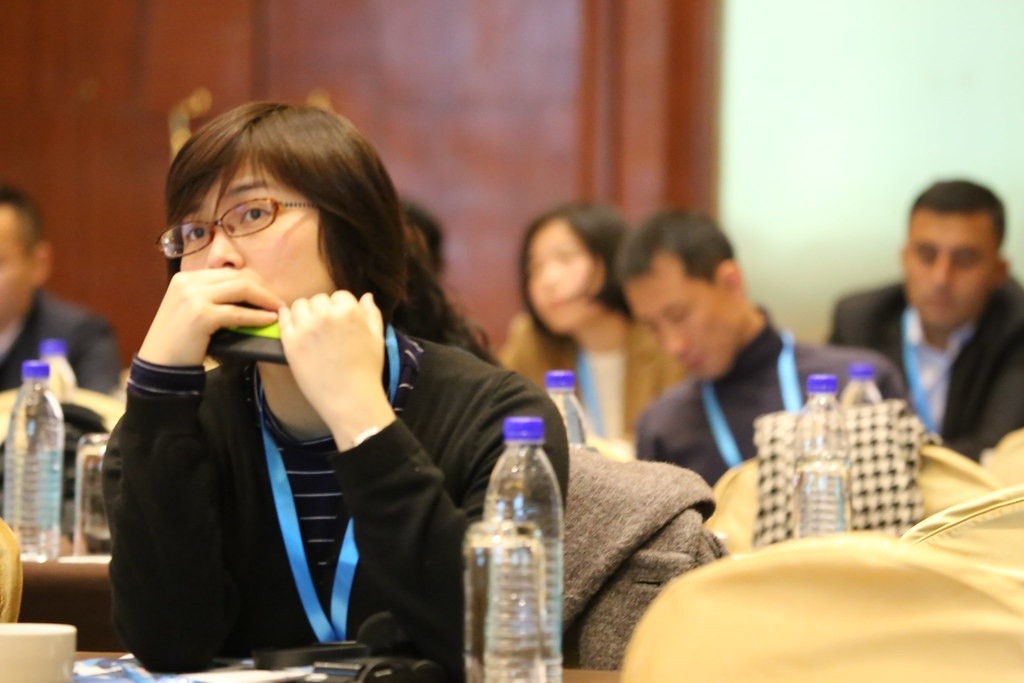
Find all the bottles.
[543,370,587,446]
[794,373,850,538]
[35,338,76,390]
[482,417,564,683]
[3,360,64,564]
[840,360,884,407]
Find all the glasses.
[155,199,319,257]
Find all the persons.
[0,180,1024,490]
[96,99,570,674]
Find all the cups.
[73,432,113,559]
[0,623,76,683]
[461,521,546,683]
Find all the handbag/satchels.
[750,392,928,545]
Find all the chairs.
[568,398,1024,683]
